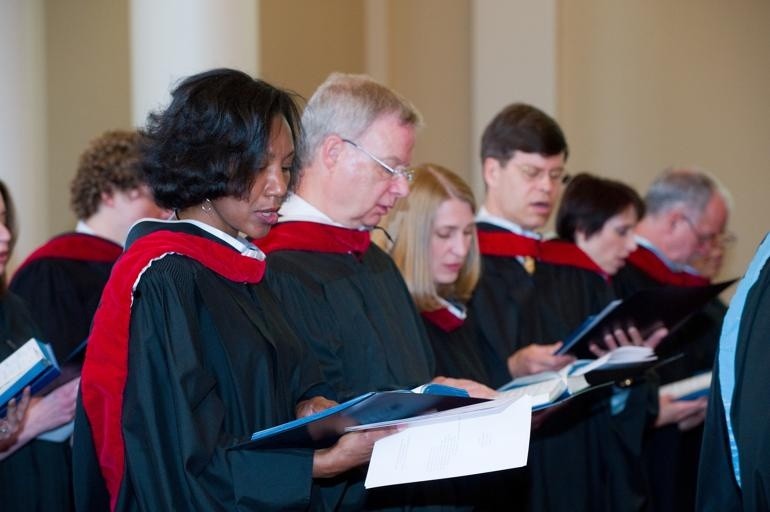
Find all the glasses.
[684,216,721,242]
[342,139,416,181]
